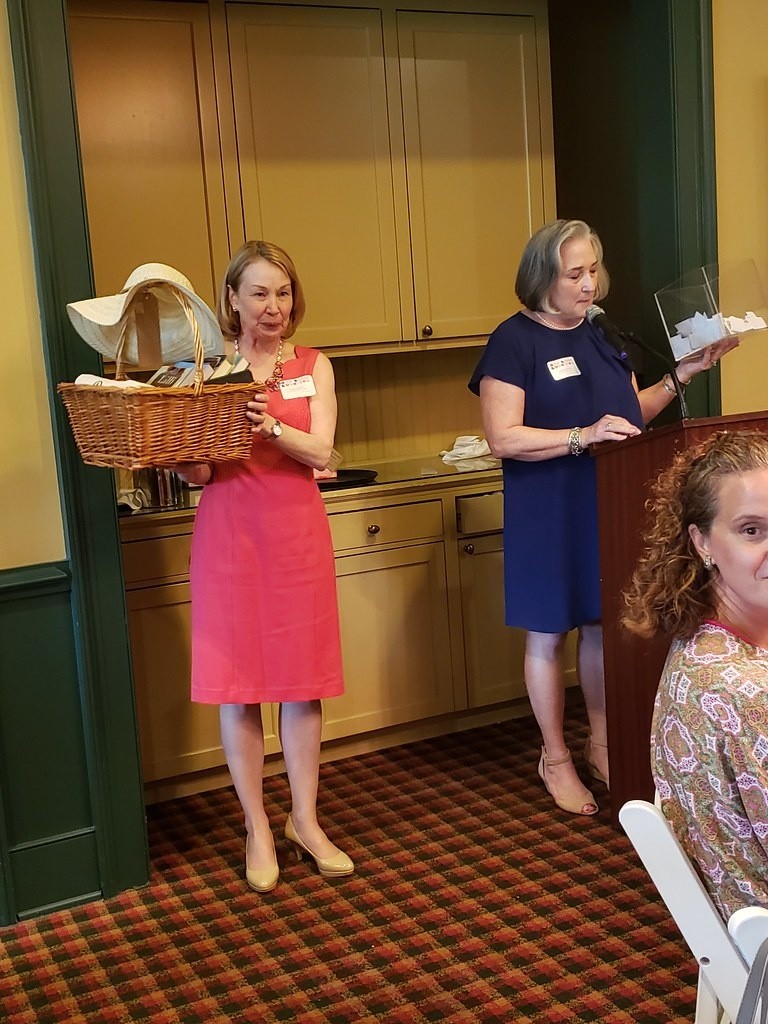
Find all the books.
[147,354,251,387]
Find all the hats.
[66,262,225,366]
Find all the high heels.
[246,829,279,893]
[284,812,354,878]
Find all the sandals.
[540,745,599,816]
[583,733,609,791]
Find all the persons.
[165,241,353,893]
[620,430,768,928]
[468,220,740,813]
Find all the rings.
[606,422,613,429]
[709,358,718,366]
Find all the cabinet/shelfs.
[66,0,557,376]
[120,446,582,805]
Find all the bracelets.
[663,373,692,395]
[568,427,584,456]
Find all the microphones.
[585,304,628,359]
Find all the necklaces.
[234,337,284,393]
[532,310,585,330]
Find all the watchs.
[262,419,284,441]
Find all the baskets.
[57,283,265,470]
